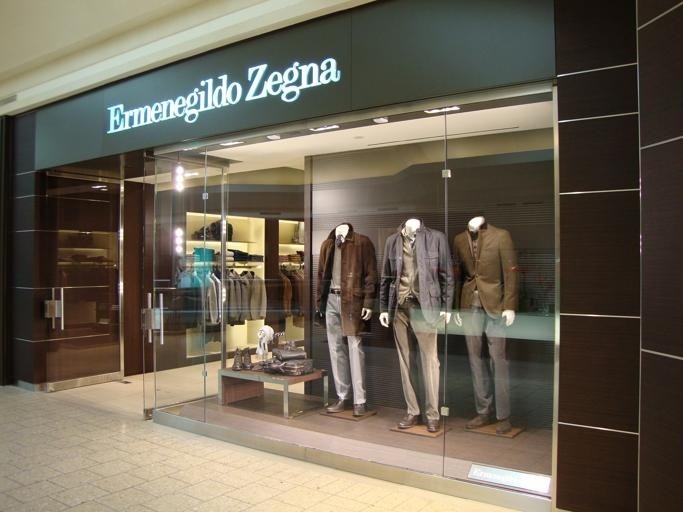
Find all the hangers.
[178,258,304,279]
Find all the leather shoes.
[325,398,344,413]
[495,416,512,435]
[397,414,423,429]
[352,403,367,417]
[426,418,441,433]
[465,414,482,429]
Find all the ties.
[336,235,343,248]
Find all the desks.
[217,363,328,419]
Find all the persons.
[451,216,519,434]
[378,215,455,431]
[315,222,378,415]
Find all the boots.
[243,346,253,370]
[231,345,243,371]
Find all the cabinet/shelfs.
[158,191,305,371]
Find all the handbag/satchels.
[211,221,233,242]
[271,345,308,362]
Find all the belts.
[329,288,341,294]
[405,297,417,303]
